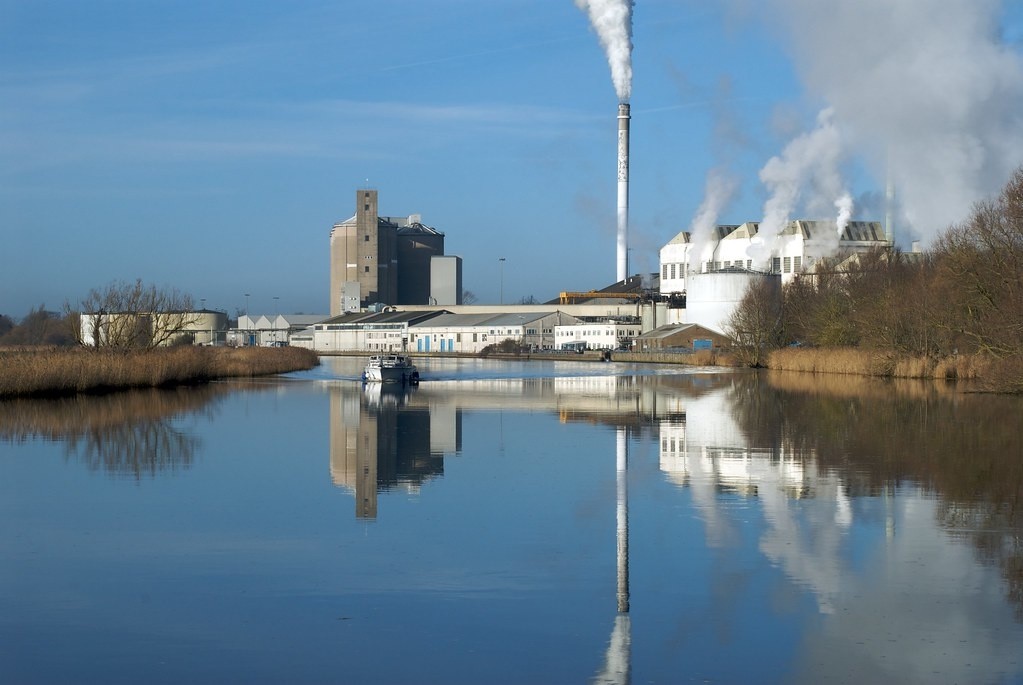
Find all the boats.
[361,349,419,382]
